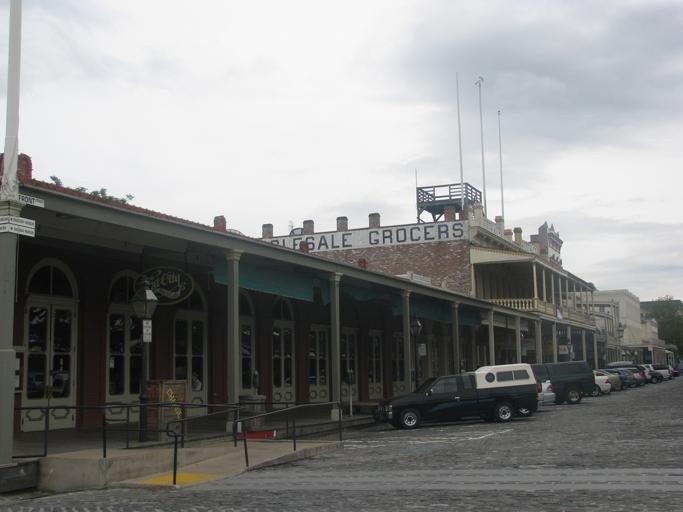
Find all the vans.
[475,360,594,406]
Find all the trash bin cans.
[239,395,266,432]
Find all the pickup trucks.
[383,373,539,430]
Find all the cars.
[591,361,678,396]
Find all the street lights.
[409,312,424,390]
[131,275,160,442]
